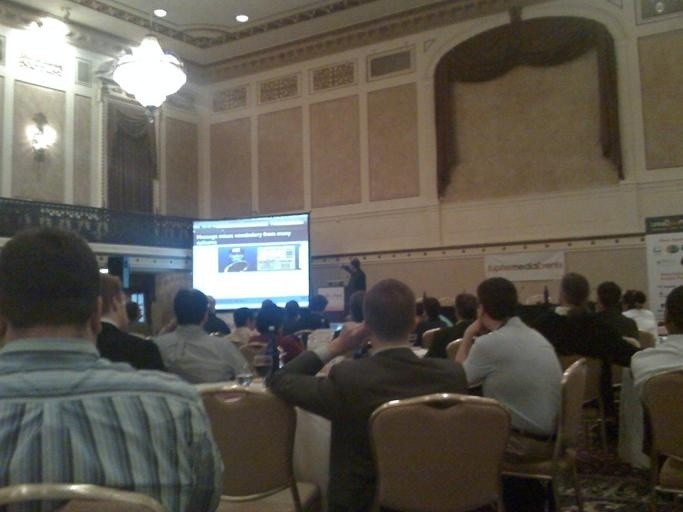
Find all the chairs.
[369,391,512,512]
[238,338,268,374]
[442,338,463,360]
[558,353,606,442]
[635,364,683,512]
[0,482,168,512]
[196,388,321,512]
[421,325,439,354]
[499,357,587,512]
[609,328,658,402]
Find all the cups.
[234,368,253,390]
[329,322,343,331]
[407,333,418,348]
[253,354,274,390]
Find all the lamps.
[20,110,57,161]
[106,31,187,124]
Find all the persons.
[282,299,301,330]
[95,272,168,372]
[227,305,262,342]
[339,257,367,294]
[125,300,154,335]
[529,272,601,358]
[411,297,447,344]
[416,302,453,328]
[204,292,231,334]
[629,283,682,505]
[149,289,257,381]
[622,289,658,344]
[444,292,477,344]
[0,225,226,512]
[587,281,640,360]
[326,289,373,355]
[304,293,330,330]
[454,275,564,511]
[266,278,471,511]
[258,298,284,333]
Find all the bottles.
[265,326,280,385]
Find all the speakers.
[109,256,130,288]
